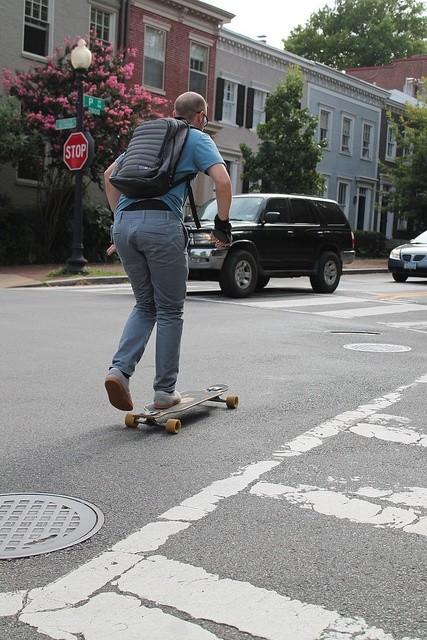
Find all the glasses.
[196,111,208,127]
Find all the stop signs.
[63,132,88,171]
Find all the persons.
[103,88,232,412]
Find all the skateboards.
[125,383,239,435]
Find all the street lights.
[64,38,93,275]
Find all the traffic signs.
[55,117,77,130]
[88,106,101,115]
[83,94,105,111]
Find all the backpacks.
[108,116,210,229]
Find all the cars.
[388,228,427,283]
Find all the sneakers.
[104,368,133,411]
[153,390,181,409]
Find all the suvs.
[184,193,356,298]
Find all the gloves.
[213,215,234,245]
